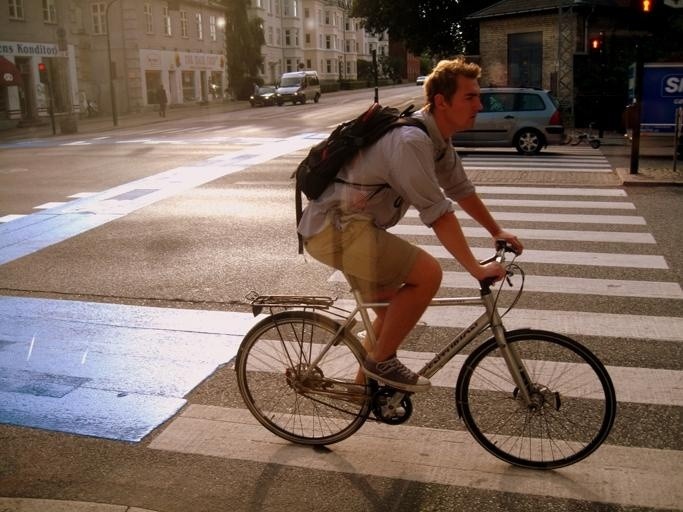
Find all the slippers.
[289,102,412,198]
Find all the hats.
[248,85,278,106]
[416,76,425,85]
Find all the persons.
[294,58,524,418]
[253,82,260,94]
[156,84,168,118]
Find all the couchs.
[590,38,604,63]
[638,0,663,18]
[38,63,47,81]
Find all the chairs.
[355,354,432,395]
[347,384,408,418]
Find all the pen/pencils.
[275,70,321,105]
[450,87,566,156]
